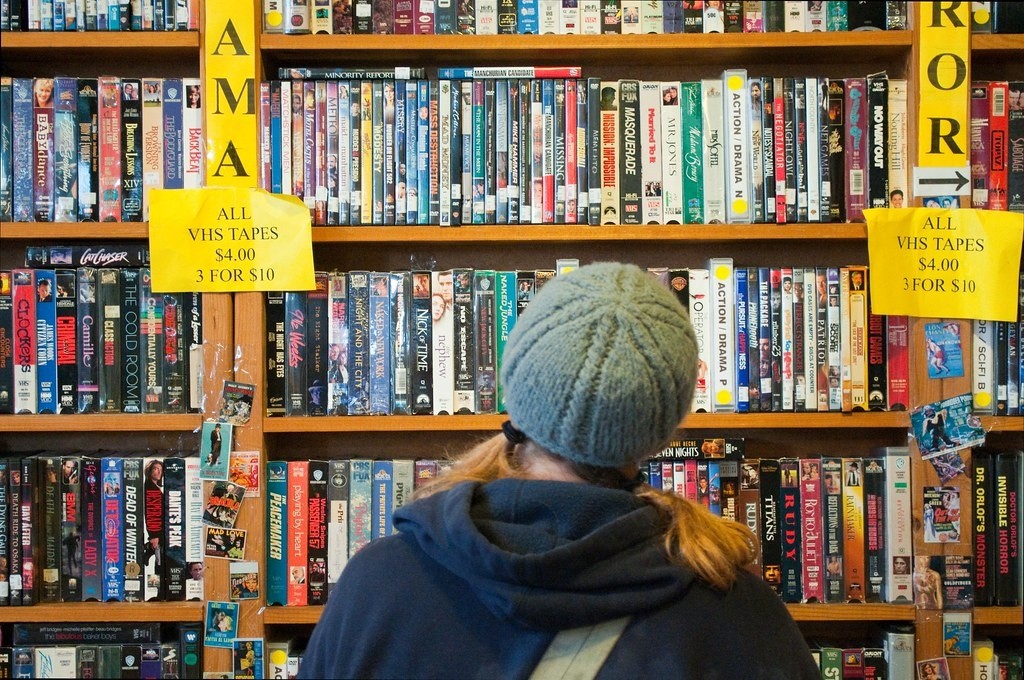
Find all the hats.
[939,486,961,498]
[503,260,699,468]
[214,423,222,429]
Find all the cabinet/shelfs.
[1,0,1023,680]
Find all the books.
[0,0,1024,224]
[1,238,913,680]
[973,272,1024,680]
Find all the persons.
[34,1,960,454]
[8,423,944,680]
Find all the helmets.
[923,405,936,415]
